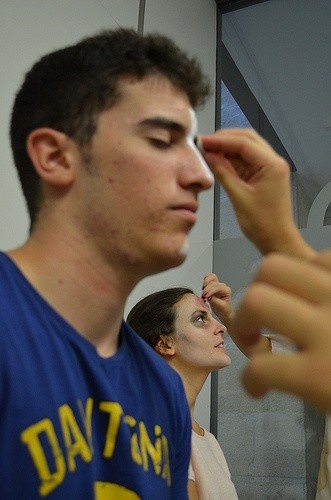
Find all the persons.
[200,273,295,364]
[126,287,240,500]
[198,126,331,414]
[0,26,214,500]
